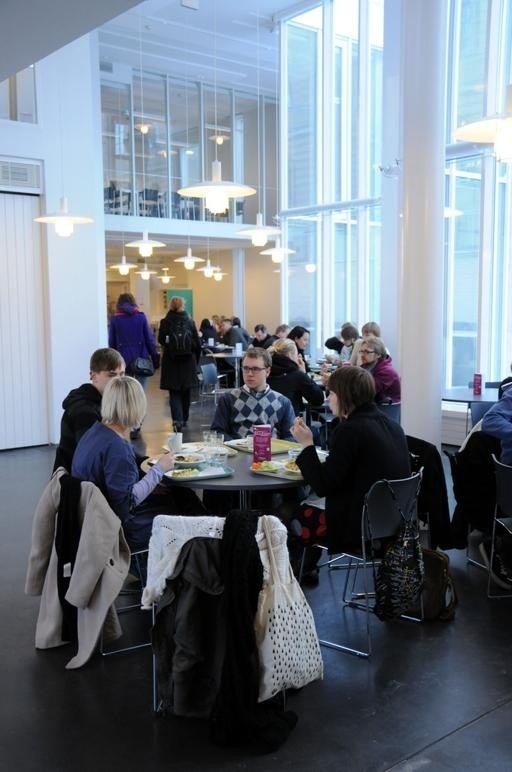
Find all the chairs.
[104,181,248,223]
[442,400,512,599]
[54,332,425,723]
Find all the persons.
[482,388,511,469]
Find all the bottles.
[473,373,482,396]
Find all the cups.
[236,342,243,355]
[202,431,225,466]
[167,433,182,452]
[207,338,215,347]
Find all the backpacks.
[162,318,193,353]
[365,474,425,621]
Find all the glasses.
[358,349,376,355]
[240,364,269,373]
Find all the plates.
[305,355,338,382]
[148,437,329,482]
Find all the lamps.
[174,50,206,270]
[32,1,94,236]
[195,80,221,278]
[178,1,256,213]
[260,16,296,262]
[110,79,138,277]
[213,210,228,282]
[237,1,281,246]
[135,264,156,280]
[156,270,175,284]
[453,82,512,165]
[127,14,166,258]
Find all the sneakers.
[294,567,320,589]
[171,419,189,434]
[129,430,141,439]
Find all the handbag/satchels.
[451,502,468,549]
[255,513,324,702]
[421,546,458,620]
[132,357,154,377]
